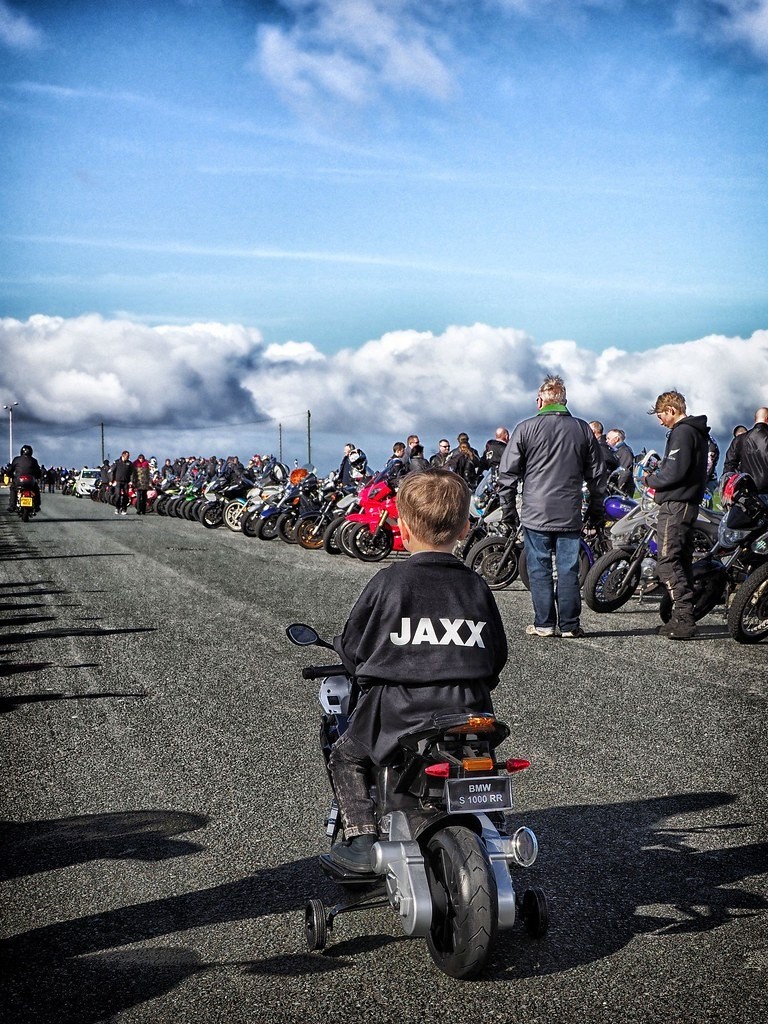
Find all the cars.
[75,469,102,498]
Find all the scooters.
[285,623,551,981]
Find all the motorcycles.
[9,475,37,522]
[61,445,767,645]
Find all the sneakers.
[562,628,585,638]
[526,625,556,637]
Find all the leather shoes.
[655,622,697,638]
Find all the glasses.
[441,445,450,448]
[410,441,419,444]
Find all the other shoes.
[137,511,141,515]
[142,511,146,515]
[114,509,119,515]
[121,510,128,515]
[329,834,375,873]
[7,506,15,513]
[34,507,41,512]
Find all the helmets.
[19,445,32,457]
[632,449,662,500]
[273,462,289,480]
[718,471,755,507]
[348,448,366,473]
[302,473,318,494]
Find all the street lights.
[4,402,18,465]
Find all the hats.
[253,454,260,460]
[261,455,269,462]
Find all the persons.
[0,444,76,514]
[162,454,269,479]
[499,376,607,637]
[339,428,509,487]
[635,391,768,640]
[589,421,636,499]
[84,451,160,515]
[328,468,509,874]
